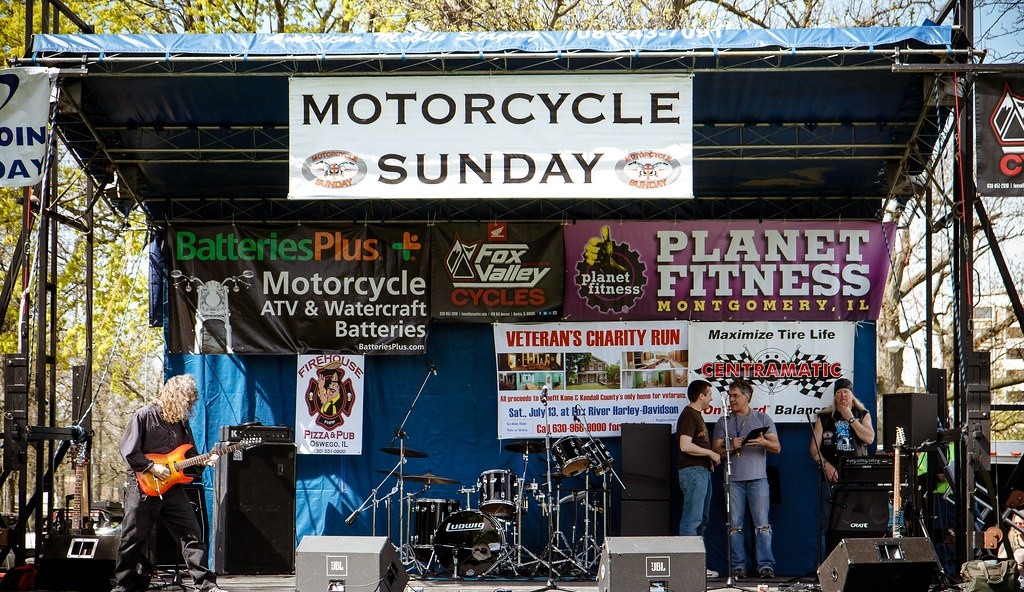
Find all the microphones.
[541,385,548,400]
[433,366,438,376]
[575,409,577,420]
[829,499,847,509]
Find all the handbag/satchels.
[960,559,1020,592]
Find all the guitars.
[55,440,97,537]
[881,426,908,539]
[134,433,264,499]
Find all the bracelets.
[817,457,829,469]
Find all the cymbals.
[380,447,430,459]
[397,473,462,485]
[374,468,408,476]
[504,441,546,454]
[559,490,594,505]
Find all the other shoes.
[194,586,228,592]
[707,569,719,577]
[111,586,125,592]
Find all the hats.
[834,379,852,397]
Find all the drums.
[432,508,506,579]
[479,468,517,516]
[583,438,615,477]
[413,498,463,548]
[551,435,591,477]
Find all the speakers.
[621,501,671,536]
[825,481,911,539]
[212,441,297,576]
[883,393,938,452]
[596,535,707,592]
[38,533,155,592]
[816,537,937,592]
[294,535,410,592]
[620,422,671,501]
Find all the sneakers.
[761,569,774,579]
[733,569,746,579]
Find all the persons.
[676,380,721,577]
[112,373,229,592]
[1008,508,1024,592]
[810,378,876,566]
[713,381,782,581]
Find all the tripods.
[481,400,631,592]
[390,368,433,579]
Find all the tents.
[22,25,969,591]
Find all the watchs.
[848,417,856,423]
